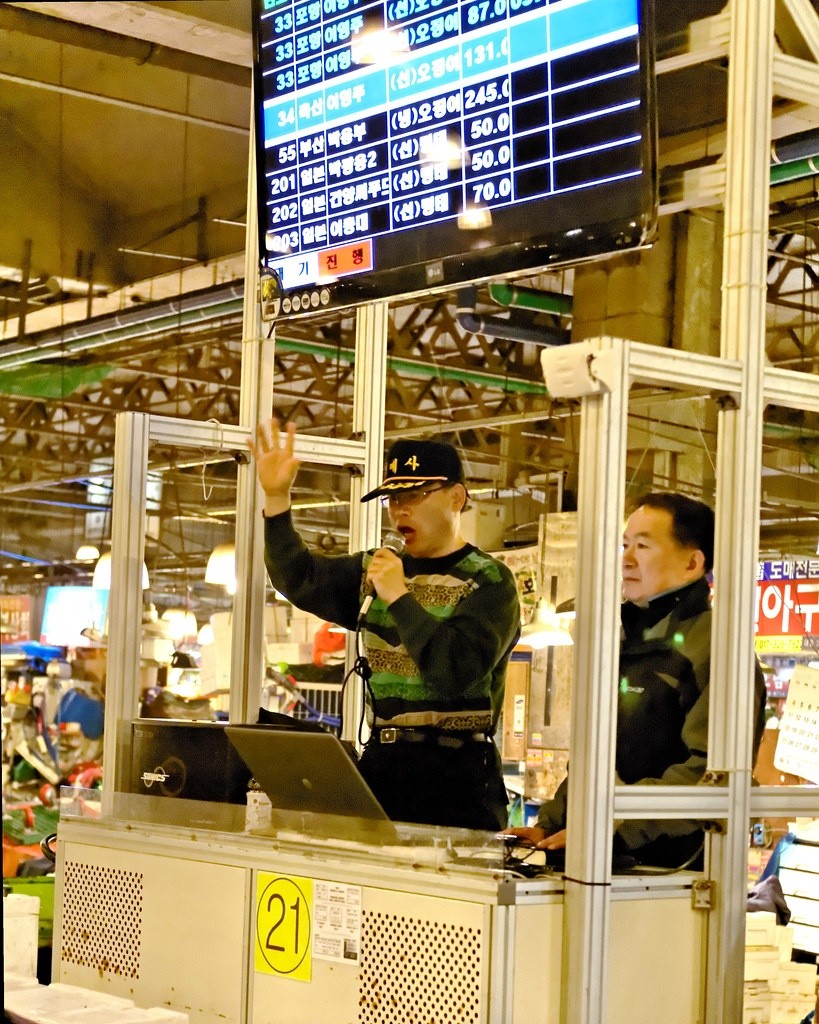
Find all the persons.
[498,493,766,872]
[244,416,522,831]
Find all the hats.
[361,440,465,502]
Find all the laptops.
[225,726,504,849]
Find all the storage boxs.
[267,641,314,665]
[742,911,818,1024]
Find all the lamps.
[205,543,236,595]
[92,551,150,591]
[556,598,576,621]
[74,545,100,564]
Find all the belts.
[372,728,486,749]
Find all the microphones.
[357,531,406,624]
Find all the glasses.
[381,485,445,509]
